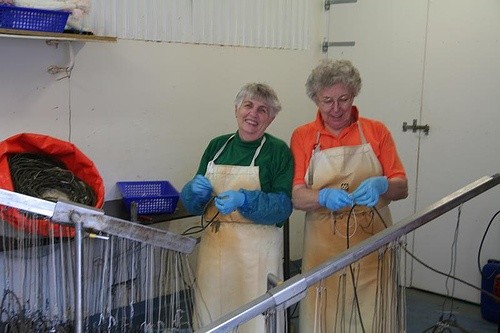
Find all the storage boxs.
[0,3,73,32]
[116,180,180,213]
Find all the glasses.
[318,94,352,106]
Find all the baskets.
[0,3,73,33]
[115,179,179,216]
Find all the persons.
[180,83,295,333]
[289,59,408,333]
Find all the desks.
[0,193,202,253]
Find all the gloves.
[316,187,352,212]
[351,174,388,208]
[190,174,215,195]
[213,190,245,216]
